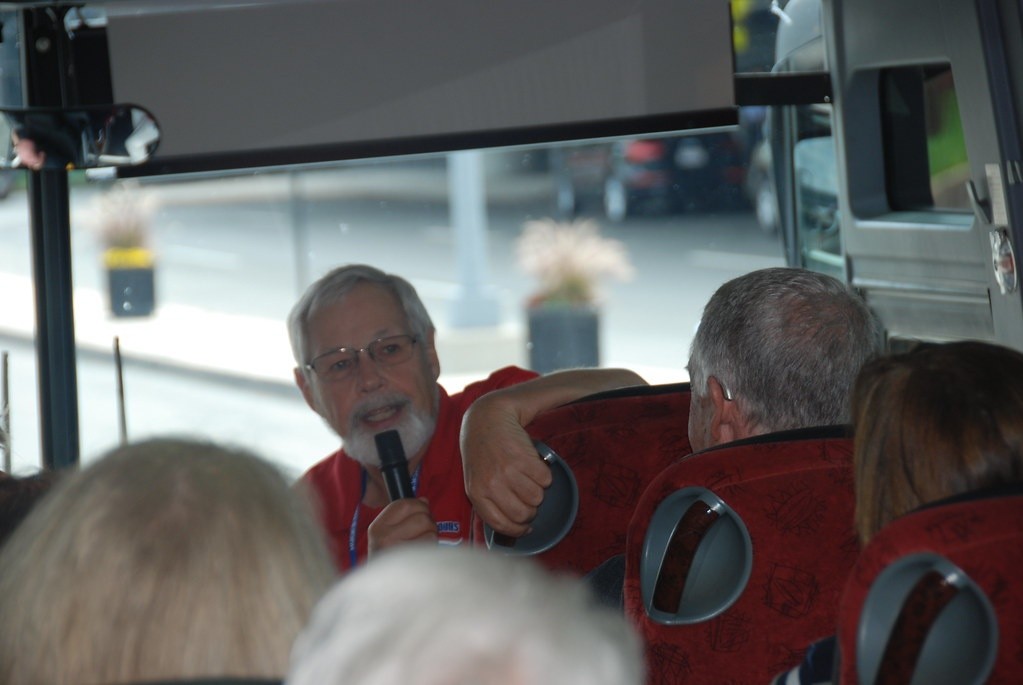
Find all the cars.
[742,105,834,234]
[544,128,743,226]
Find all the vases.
[108,265,155,317]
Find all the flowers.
[101,245,158,269]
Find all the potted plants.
[514,216,636,376]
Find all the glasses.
[306,335,417,383]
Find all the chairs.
[621,421,861,685]
[836,486,1023,685]
[468,382,692,614]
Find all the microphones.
[375,430,415,504]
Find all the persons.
[9,126,65,170]
[0,262,1023,685]
